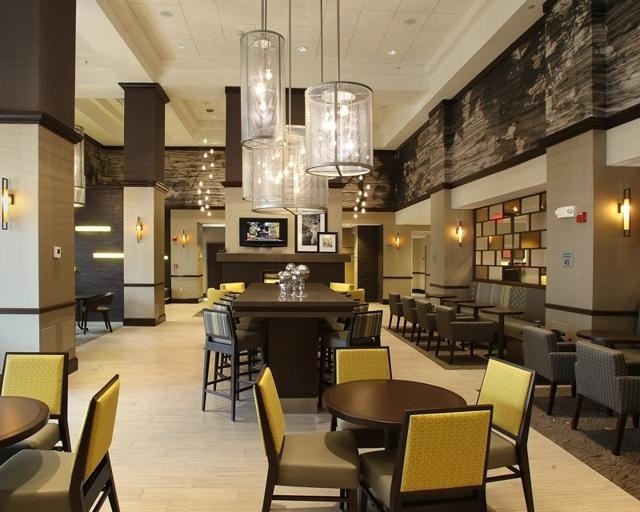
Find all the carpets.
[475,387,640,501]
[380,326,488,370]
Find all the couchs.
[461,281,547,342]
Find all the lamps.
[251,0,329,215]
[136,217,142,243]
[74,124,87,208]
[181,230,186,247]
[353,175,371,219]
[618,188,631,237]
[93,252,169,260]
[197,109,215,216]
[239,16,288,149]
[395,232,400,251]
[304,17,373,178]
[456,221,463,248]
[0,177,14,230]
[75,225,111,232]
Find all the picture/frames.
[295,208,328,253]
[317,232,338,253]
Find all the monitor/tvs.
[239,217,287,247]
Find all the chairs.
[402,295,436,342]
[330,346,392,448]
[416,300,468,352]
[357,403,494,512]
[84,291,115,335]
[522,325,576,416]
[202,282,266,422]
[388,292,404,333]
[320,282,384,385]
[0,352,73,453]
[476,356,538,512]
[435,305,497,365]
[253,364,361,512]
[0,374,121,512]
[570,340,640,456]
